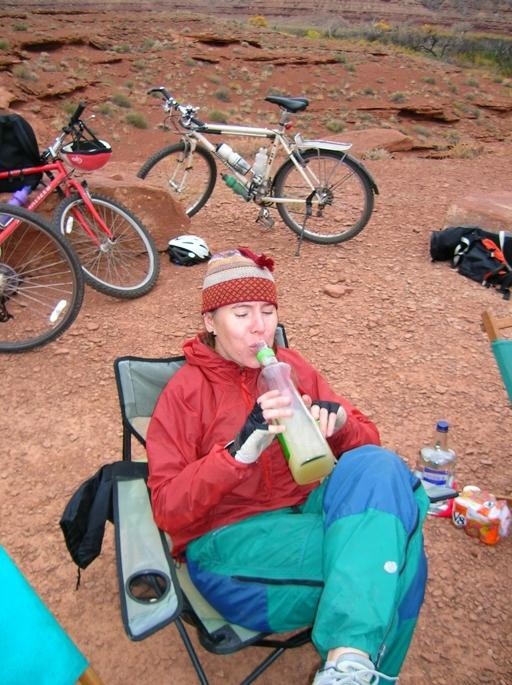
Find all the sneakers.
[311,650,401,685]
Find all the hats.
[199,246,283,317]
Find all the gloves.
[304,398,351,441]
[223,400,281,466]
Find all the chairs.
[112,322,315,684]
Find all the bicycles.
[0,100,158,353]
[135,87,380,258]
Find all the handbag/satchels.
[450,229,512,301]
[0,112,43,193]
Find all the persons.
[144,246,433,685]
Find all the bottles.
[411,420,458,519]
[0,184,33,230]
[254,338,337,487]
[219,172,254,203]
[252,146,270,180]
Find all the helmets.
[165,233,210,260]
[59,139,113,173]
